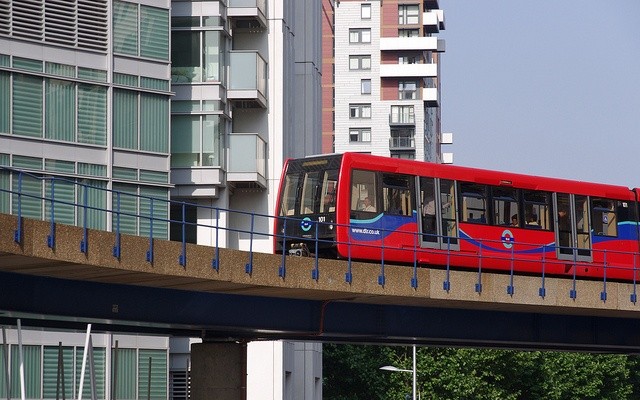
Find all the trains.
[273,152,640,283]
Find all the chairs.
[536,225,542,230]
[525,224,536,229]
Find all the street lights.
[380,347,416,400]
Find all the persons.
[529,214,538,225]
[558,208,571,254]
[363,197,376,212]
[510,214,518,226]
[423,193,452,242]
